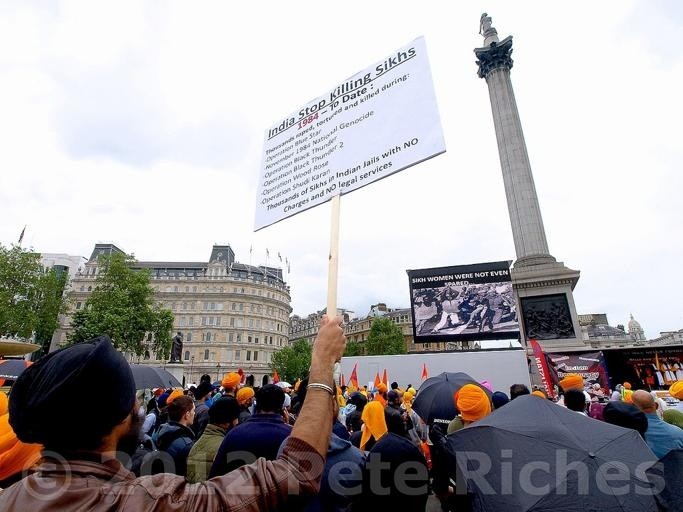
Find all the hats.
[195,382,216,400]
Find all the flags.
[277,251,281,261]
[249,245,251,253]
[17,228,24,242]
[266,249,268,257]
[288,262,290,274]
[284,257,287,265]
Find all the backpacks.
[130,424,191,477]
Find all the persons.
[1,312,683,511]
[412,283,518,337]
